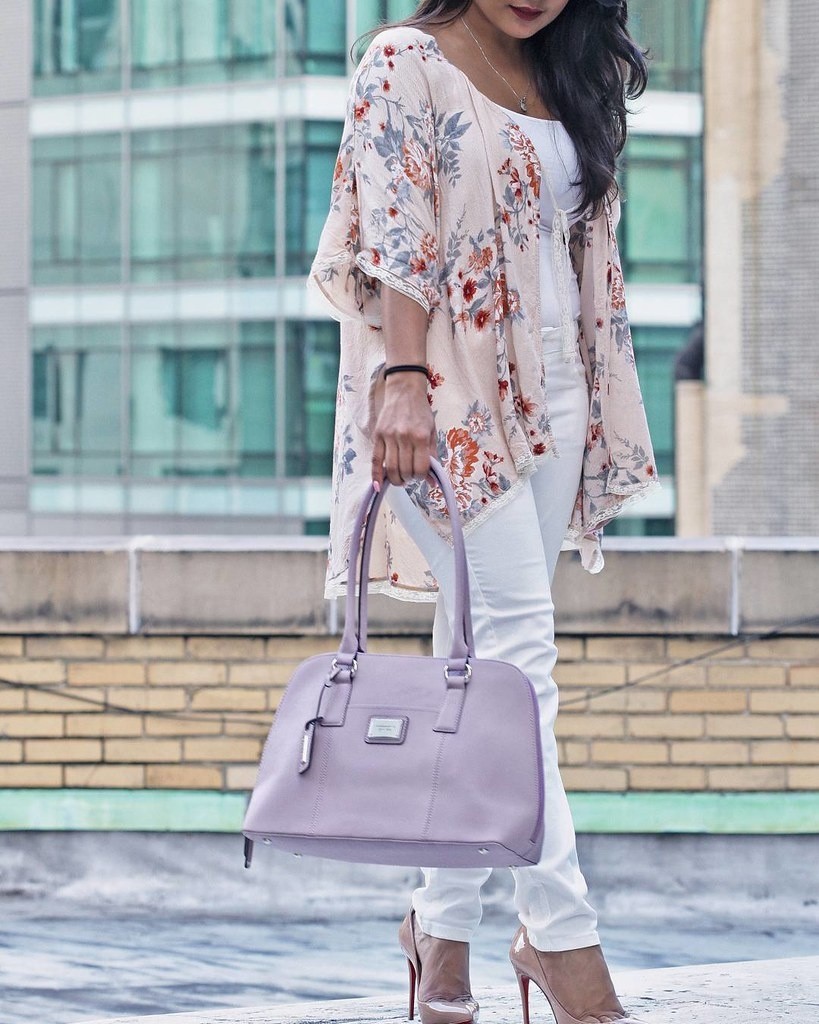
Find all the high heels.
[509,921,636,1023]
[398,907,478,1024]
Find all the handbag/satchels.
[241,453,545,871]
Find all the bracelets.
[384,365,428,380]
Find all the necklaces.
[458,9,531,111]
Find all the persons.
[307,0,658,1024]
